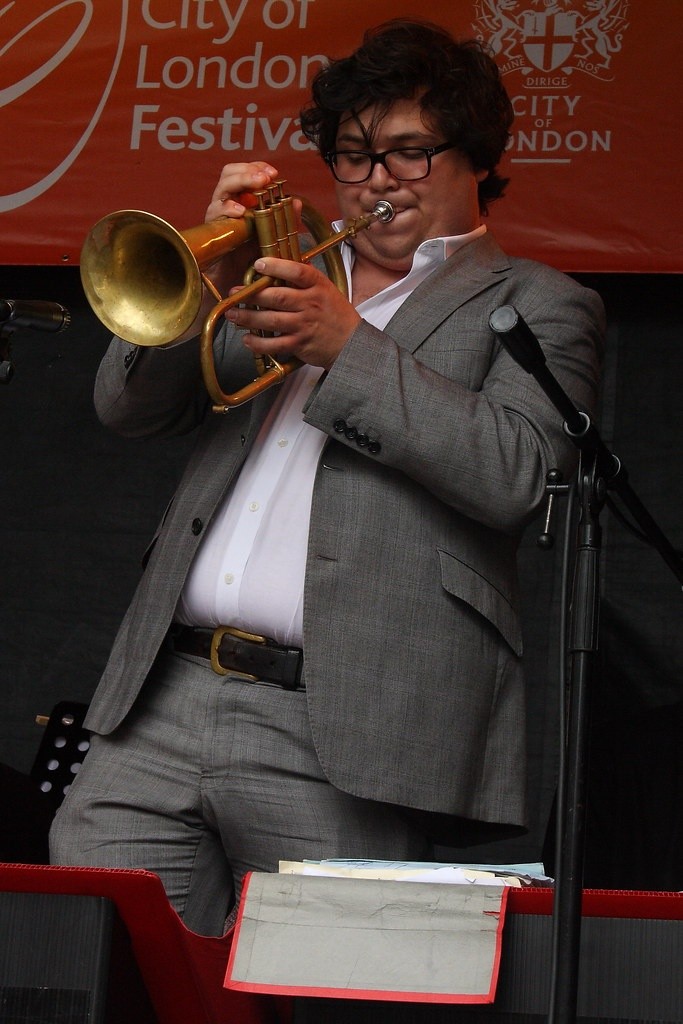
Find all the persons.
[51,15,611,936]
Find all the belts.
[167,620,306,689]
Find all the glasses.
[324,137,467,182]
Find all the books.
[279,856,554,887]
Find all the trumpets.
[77,177,398,417]
